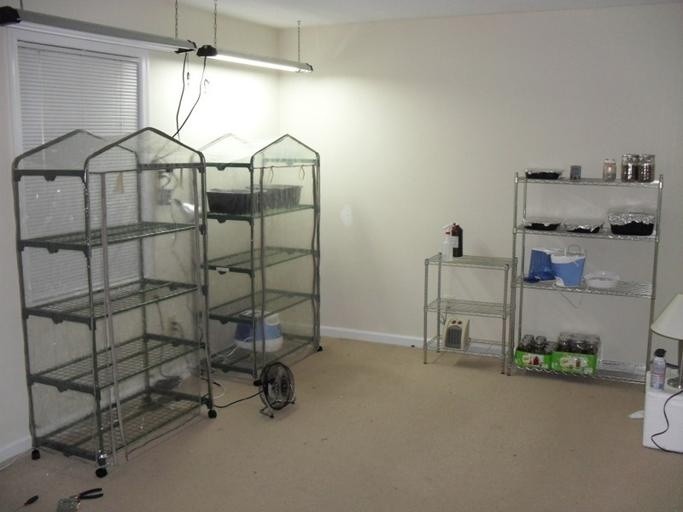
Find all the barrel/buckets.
[550,243,585,287]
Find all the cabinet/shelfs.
[425,253,518,374]
[510,169,663,386]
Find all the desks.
[640,371,682,454]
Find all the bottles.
[557,330,600,354]
[621,153,656,182]
[442,222,464,263]
[519,332,547,354]
[651,348,668,392]
[602,158,617,183]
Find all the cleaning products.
[442,224,454,262]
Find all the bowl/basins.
[584,271,621,289]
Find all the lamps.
[197,0,315,72]
[1,0,197,54]
[651,293,682,391]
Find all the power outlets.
[168,316,181,338]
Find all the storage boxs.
[550,349,598,377]
[514,343,552,371]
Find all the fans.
[252,361,296,419]
